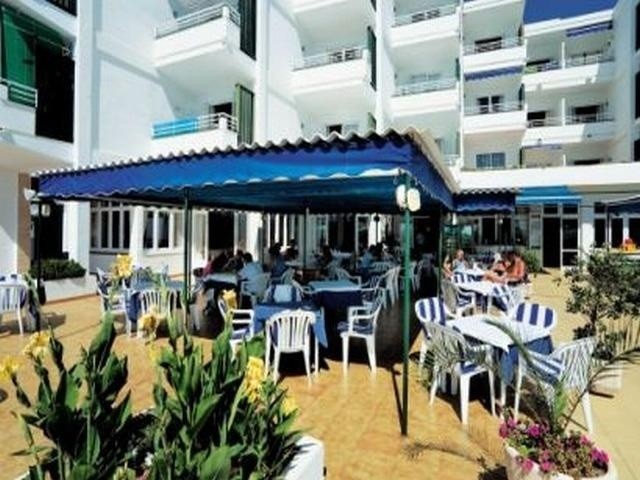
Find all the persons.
[286,247,305,283]
[481,251,526,285]
[233,250,244,270]
[441,250,468,275]
[210,246,234,302]
[315,243,337,281]
[361,239,401,261]
[262,243,282,272]
[236,252,264,302]
[289,239,298,249]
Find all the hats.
[493,253,503,261]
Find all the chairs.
[508,301,557,330]
[340,288,387,373]
[427,323,497,426]
[516,336,599,435]
[414,298,458,380]
[1,282,27,335]
[265,310,317,387]
